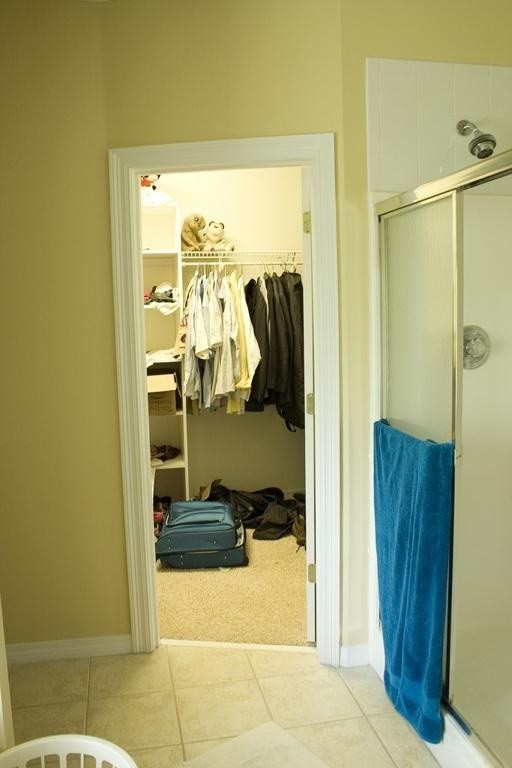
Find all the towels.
[373,417,455,746]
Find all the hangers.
[188,259,297,293]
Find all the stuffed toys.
[180,214,236,256]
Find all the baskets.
[1,733,138,768]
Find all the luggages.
[155,501,249,569]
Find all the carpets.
[176,721,331,768]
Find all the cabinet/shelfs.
[141,203,189,520]
